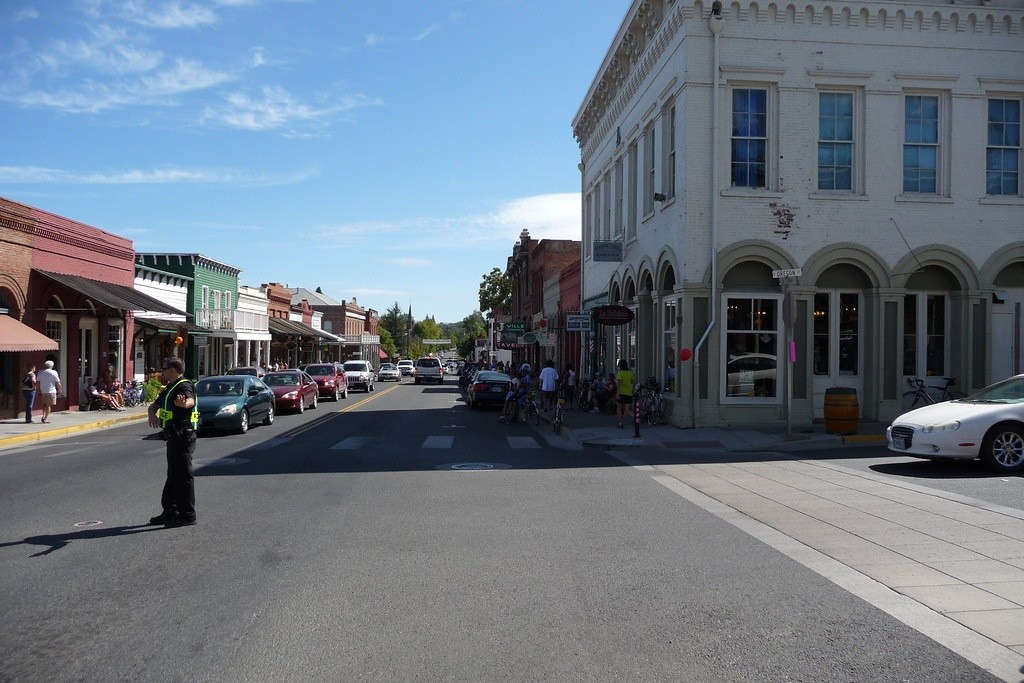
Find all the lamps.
[139,338,145,345]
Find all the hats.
[45,361,54,367]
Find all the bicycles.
[901,375,970,413]
[636,377,669,427]
[575,377,592,411]
[552,398,565,435]
[519,394,540,426]
[502,391,517,426]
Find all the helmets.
[521,364,531,372]
[636,382,643,392]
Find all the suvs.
[344,360,376,392]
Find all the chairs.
[603,392,618,413]
[84,389,102,410]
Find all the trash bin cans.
[754,379,776,398]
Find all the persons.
[489,359,617,420]
[37,360,63,424]
[617,359,638,429]
[20,364,37,424]
[86,377,127,413]
[147,356,200,529]
[291,376,300,385]
[234,383,242,392]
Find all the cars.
[886,374,1024,473]
[302,362,349,401]
[413,357,446,385]
[397,360,415,377]
[439,351,480,399]
[726,353,777,398]
[263,364,319,415]
[378,363,402,382]
[223,366,267,381]
[466,370,513,410]
[193,375,277,434]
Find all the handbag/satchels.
[22,374,33,388]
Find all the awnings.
[269,317,347,344]
[134,317,214,347]
[0,313,60,353]
[27,267,194,318]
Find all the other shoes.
[165,512,196,527]
[616,422,624,430]
[150,510,179,524]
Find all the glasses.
[160,368,170,371]
